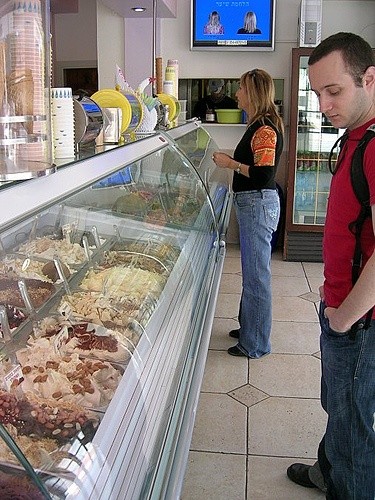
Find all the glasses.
[249,69,258,89]
[329,132,348,175]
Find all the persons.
[161,148,200,218]
[192,80,238,119]
[212,68,285,359]
[203,10,224,34]
[237,11,262,34]
[287,31,375,500]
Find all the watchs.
[236,163,242,174]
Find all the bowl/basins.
[46,87,76,159]
[180,100,188,110]
[179,112,187,122]
[215,109,243,123]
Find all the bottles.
[298,151,322,171]
[205,108,215,123]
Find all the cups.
[162,59,179,124]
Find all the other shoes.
[228,346,245,356]
[287,463,318,488]
[229,330,240,338]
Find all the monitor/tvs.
[191,0,276,52]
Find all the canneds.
[102,107,122,144]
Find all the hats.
[208,79,225,93]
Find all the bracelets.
[177,202,184,206]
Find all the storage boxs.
[178,100,187,124]
